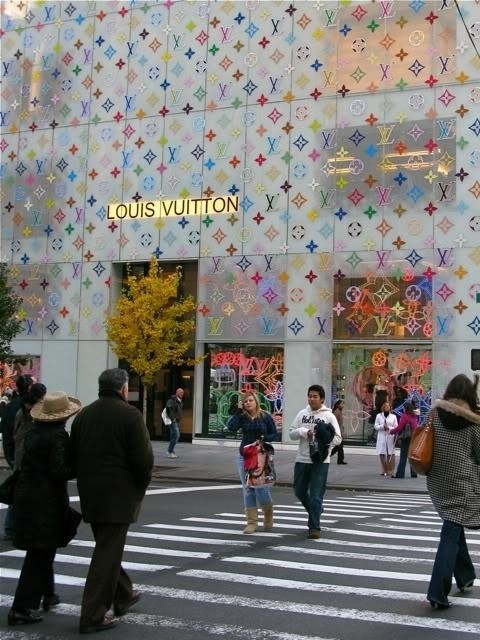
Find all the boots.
[243,506,259,535]
[261,503,273,532]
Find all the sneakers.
[337,459,349,466]
[308,528,321,539]
[167,453,179,459]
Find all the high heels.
[459,581,474,593]
[429,599,453,610]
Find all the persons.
[69,368,154,634]
[161,388,184,458]
[370,374,391,427]
[1,374,37,467]
[5,391,82,626]
[408,373,479,609]
[374,402,398,476]
[388,402,418,478]
[15,383,43,474]
[289,385,343,539]
[330,400,348,465]
[227,392,279,533]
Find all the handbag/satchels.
[161,408,172,426]
[399,413,411,440]
[246,443,276,489]
[0,466,21,505]
[408,410,436,475]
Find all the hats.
[30,390,83,422]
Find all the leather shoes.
[42,593,63,611]
[79,616,121,633]
[114,591,143,617]
[7,609,46,624]
